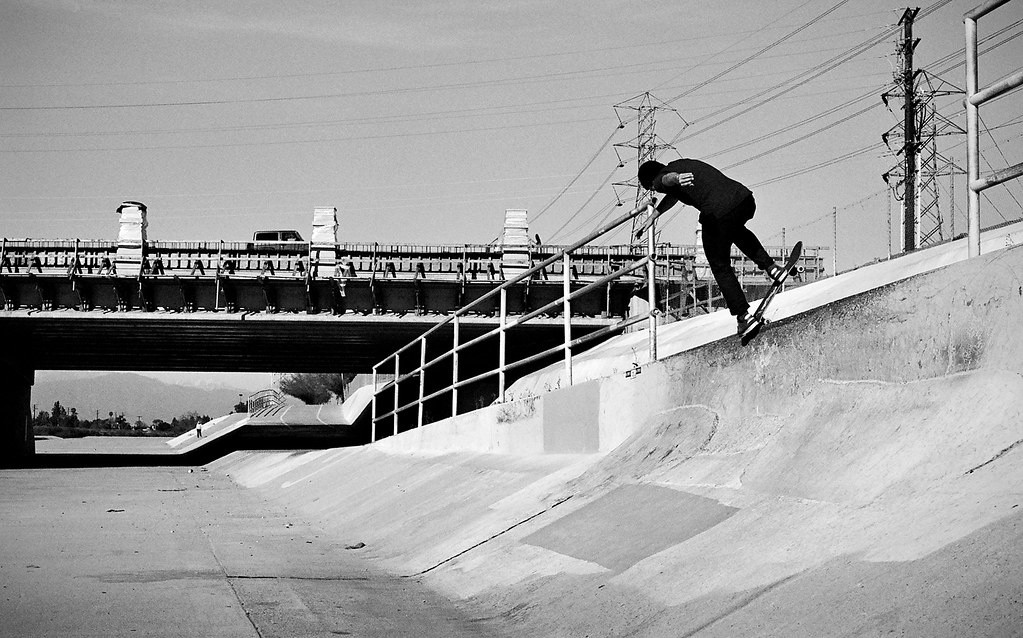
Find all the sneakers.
[766,263,788,282]
[736,311,759,337]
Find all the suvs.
[247,229,311,255]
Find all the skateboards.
[737,240,805,342]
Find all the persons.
[196,421,203,438]
[635,159,788,337]
